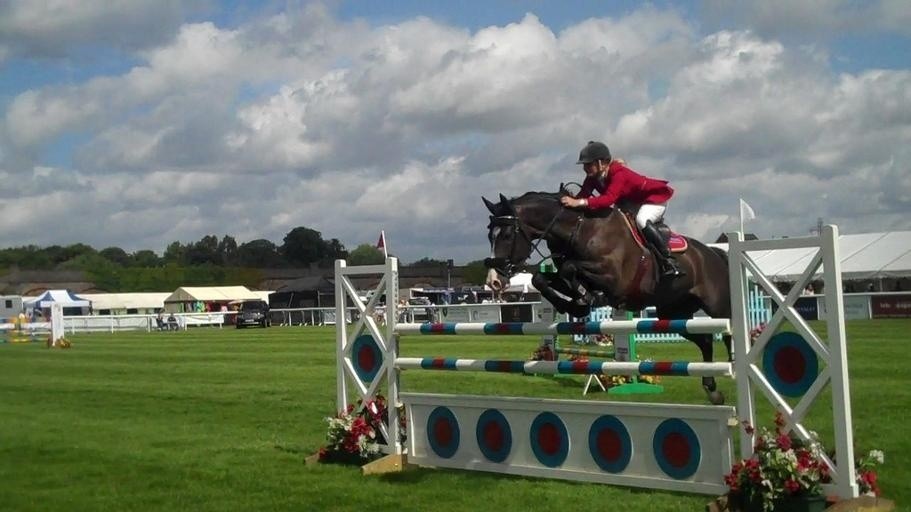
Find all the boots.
[642,220,687,281]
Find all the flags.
[374,229,385,251]
[738,196,756,221]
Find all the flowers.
[725,428,823,511]
[348,394,406,445]
[324,416,378,460]
[777,413,883,496]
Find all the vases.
[336,450,367,466]
[780,497,826,512]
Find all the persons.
[806,285,815,296]
[398,300,407,324]
[167,312,180,332]
[156,314,169,331]
[427,296,437,324]
[19,310,26,320]
[560,141,688,284]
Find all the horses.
[481,183,732,404]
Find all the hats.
[576,141,610,164]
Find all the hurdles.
[303,223,896,511]
[522,264,664,396]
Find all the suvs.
[236,300,272,328]
